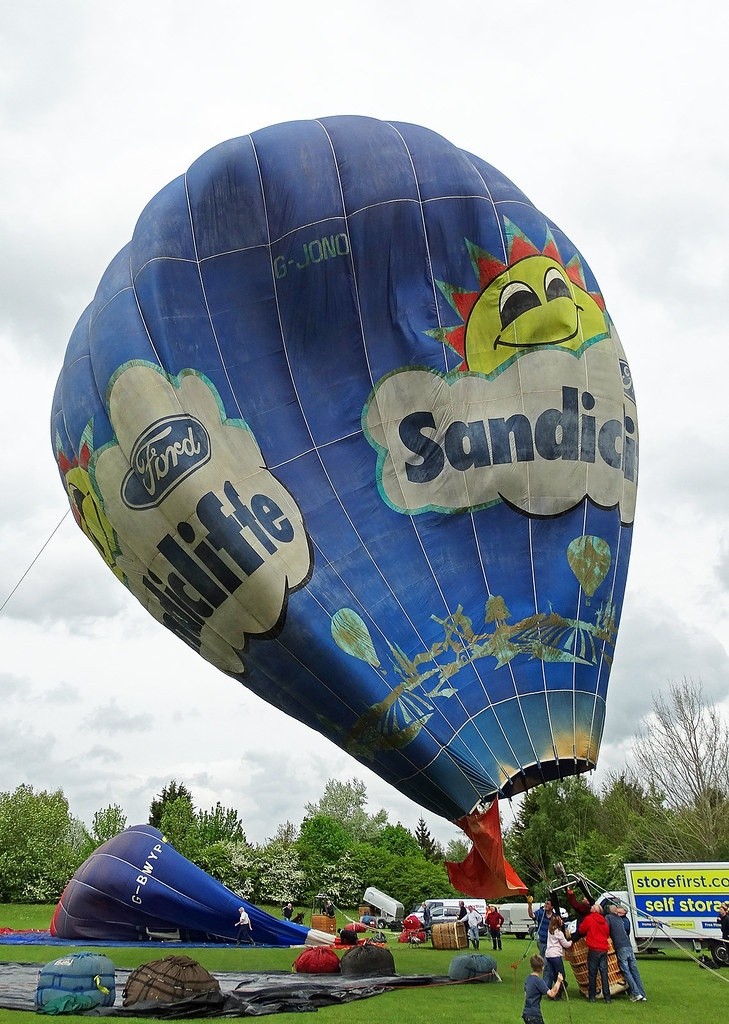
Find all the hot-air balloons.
[47,114,635,1001]
[49,824,390,949]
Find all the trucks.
[592,862,729,968]
[360,886,407,929]
[486,901,571,941]
[404,898,489,936]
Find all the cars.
[418,905,488,936]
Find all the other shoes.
[587,999,596,1002]
[630,994,643,1002]
[642,998,647,1002]
[603,1000,611,1004]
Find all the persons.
[458,905,482,950]
[456,901,470,949]
[484,906,505,950]
[281,903,293,922]
[716,903,729,938]
[322,901,334,916]
[522,955,563,1024]
[579,904,612,1004]
[526,886,595,1001]
[235,907,256,946]
[617,906,631,941]
[337,928,358,945]
[604,905,647,1003]
[422,902,432,941]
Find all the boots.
[548,987,564,1001]
[249,938,256,946]
[236,940,241,946]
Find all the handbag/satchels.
[698,954,719,969]
